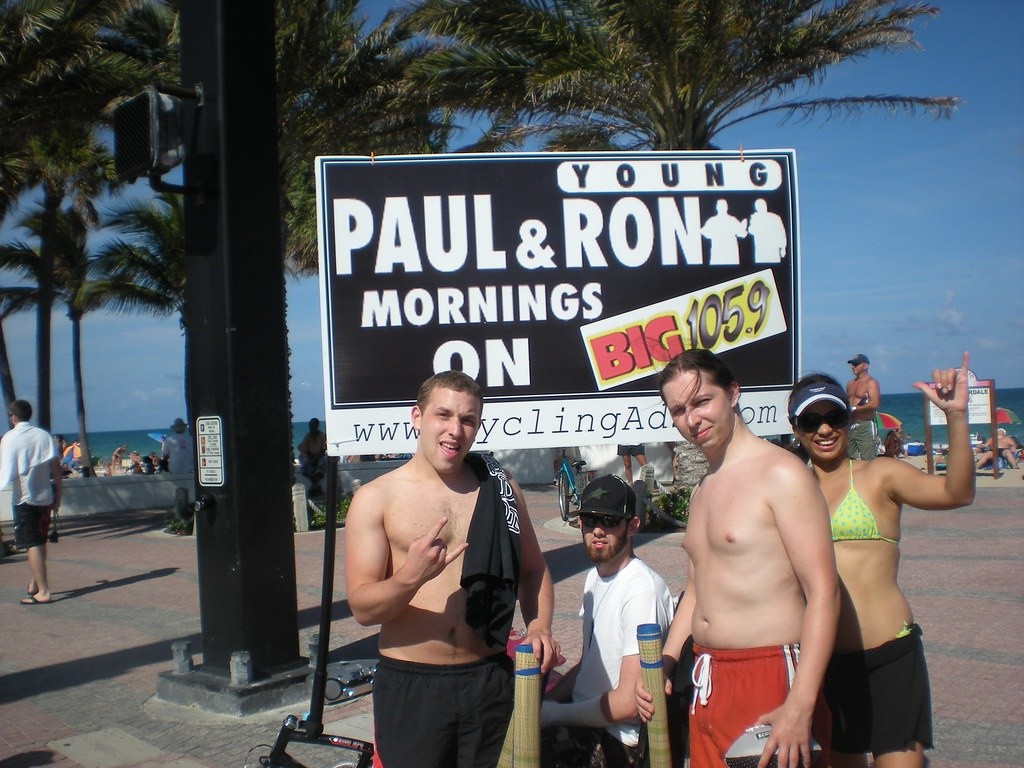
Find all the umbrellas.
[995,407,1021,425]
[63,442,81,458]
[876,411,903,430]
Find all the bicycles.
[554,447,598,521]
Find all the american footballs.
[723,724,823,768]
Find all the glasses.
[852,362,863,368]
[790,409,849,433]
[579,512,632,528]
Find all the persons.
[81,466,93,477]
[777,349,977,768]
[975,428,1020,469]
[121,451,141,473]
[0,399,63,605]
[344,370,562,768]
[62,462,68,469]
[142,456,155,474]
[1008,434,1023,457]
[885,426,912,460]
[112,445,127,470]
[845,354,880,461]
[539,472,674,768]
[162,418,195,473]
[54,434,67,453]
[635,336,842,768]
[297,417,327,497]
[618,444,648,486]
[662,441,680,485]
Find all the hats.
[575,474,635,518]
[997,427,1006,436]
[170,418,186,432]
[789,383,848,424]
[848,354,870,364]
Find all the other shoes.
[309,485,322,497]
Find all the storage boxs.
[907,443,925,455]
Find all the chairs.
[985,447,1023,470]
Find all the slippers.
[20,596,53,604]
[28,584,38,595]
